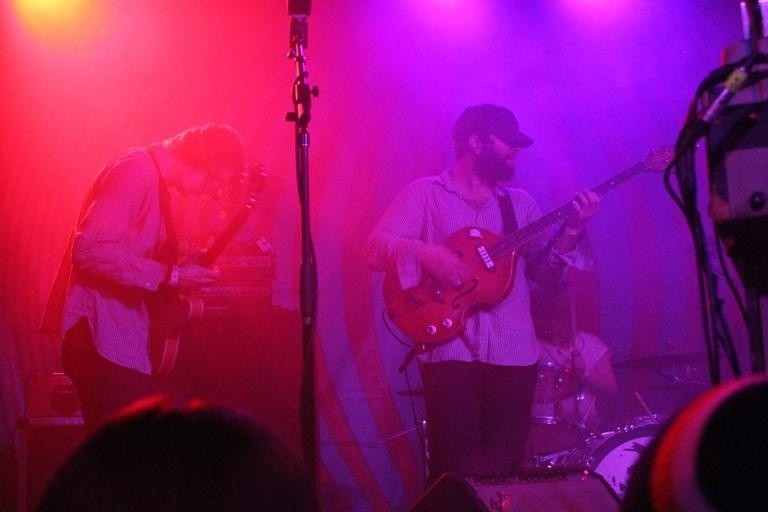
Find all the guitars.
[147,163,265,379]
[381,142,677,345]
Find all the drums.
[554,424,662,499]
[516,416,563,477]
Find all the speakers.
[406,471,623,512]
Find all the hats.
[451,104,534,148]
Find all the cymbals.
[533,361,579,403]
[610,352,708,369]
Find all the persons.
[354,102,602,485]
[618,371,768,512]
[37,393,315,512]
[37,123,258,425]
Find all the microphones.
[288,0,312,50]
